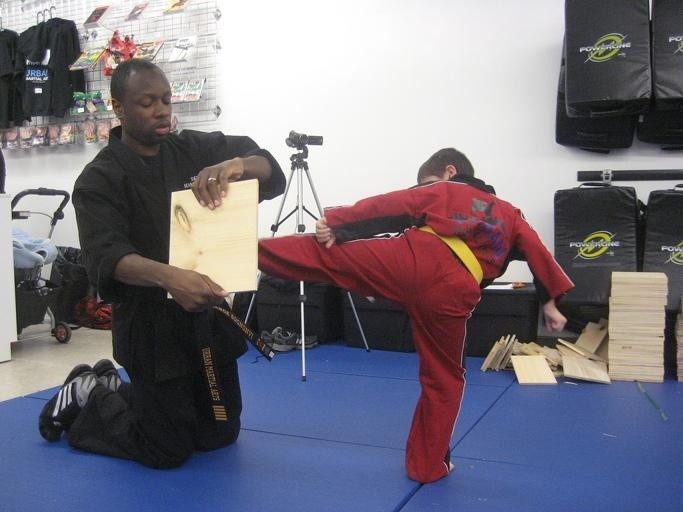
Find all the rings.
[206,177,217,183]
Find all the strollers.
[9,187,73,343]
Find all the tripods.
[242,159,374,381]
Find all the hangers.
[0,17,5,32]
[37,6,56,25]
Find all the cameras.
[285,130,323,160]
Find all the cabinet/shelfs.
[0,193,17,363]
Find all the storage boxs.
[342,291,416,352]
[257,276,341,344]
[464,283,540,358]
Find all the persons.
[258,147,575,483]
[37,57,287,469]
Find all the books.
[162,0,189,15]
[124,2,149,22]
[83,5,111,28]
[68,46,106,71]
[167,36,198,64]
[132,41,164,61]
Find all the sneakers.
[39,359,122,442]
